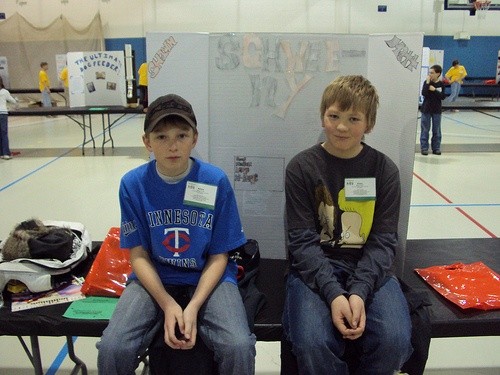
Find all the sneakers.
[0,155,11,159]
[433,149,441,155]
[421,150,428,155]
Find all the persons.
[38,62,148,108]
[420,65,446,155]
[281,75,415,375]
[96,94,256,375]
[0,76,18,160]
[441,60,467,112]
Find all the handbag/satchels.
[80,227,133,297]
[228,239,261,272]
[0,221,92,301]
[413,260,500,311]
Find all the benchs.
[0,259,291,375]
[401,237,500,375]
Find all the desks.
[6,89,67,106]
[7,106,148,155]
[444,83,500,101]
[464,77,496,80]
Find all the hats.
[144,94,197,134]
